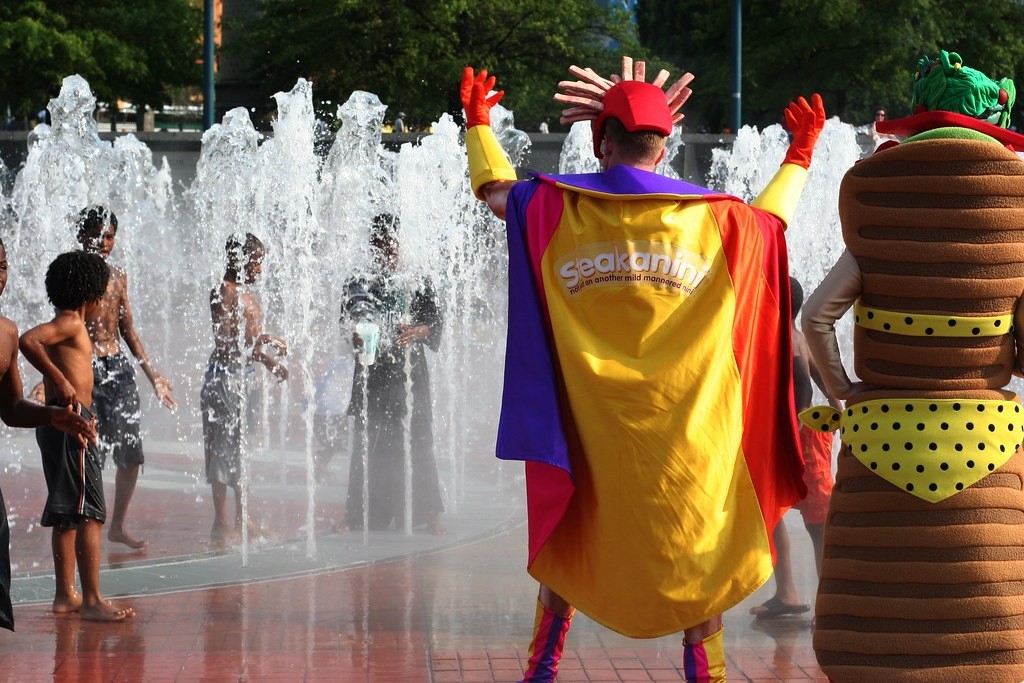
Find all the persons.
[457,56,845,683]
[335,213,448,536]
[1,203,177,631]
[201,232,288,549]
[872,107,887,124]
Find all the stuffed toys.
[797,47,1024,682]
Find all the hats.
[553,56,695,165]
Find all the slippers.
[757,597,810,620]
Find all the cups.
[356,323,379,366]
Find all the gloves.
[780,93,826,170]
[459,66,504,128]
